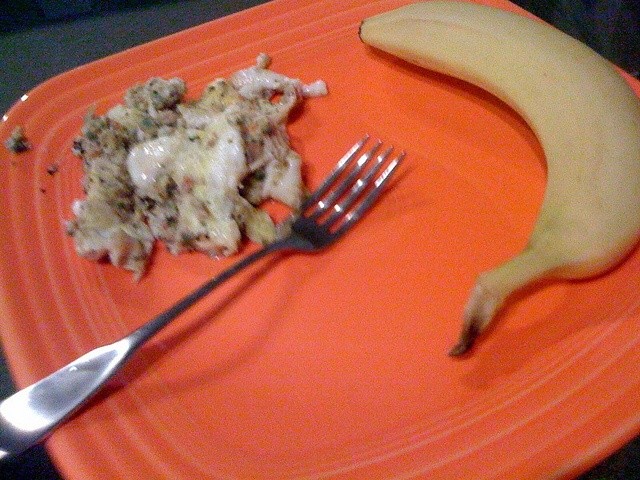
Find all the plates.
[0,0,640,480]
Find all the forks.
[0,130,406,464]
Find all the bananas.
[358,1,640,357]
